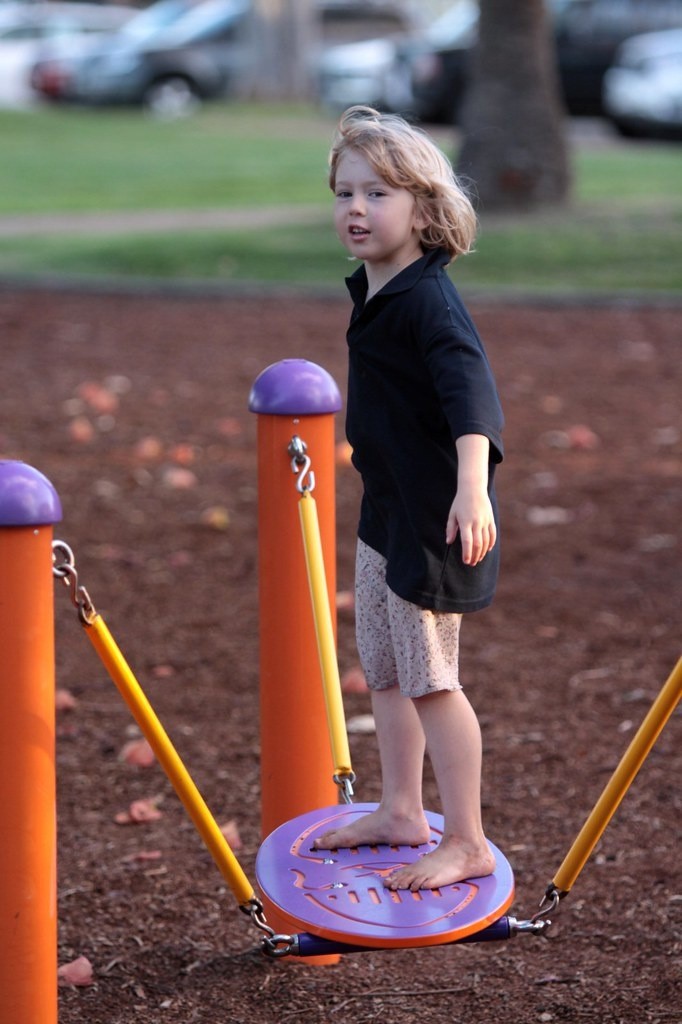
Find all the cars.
[0,1,681,139]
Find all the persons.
[310,102,507,896]
